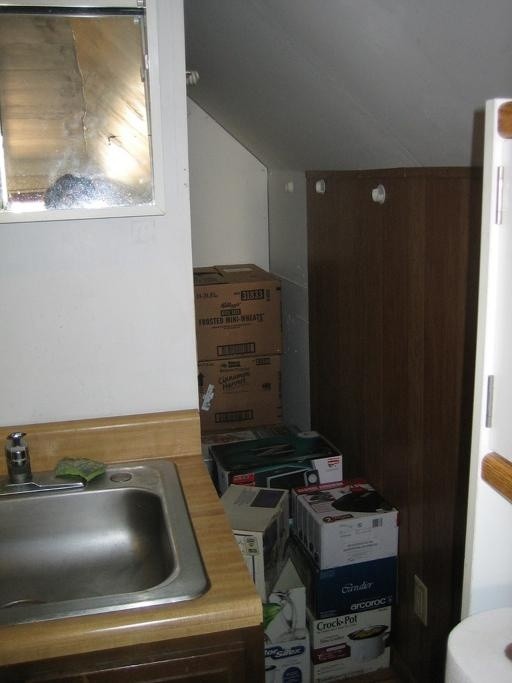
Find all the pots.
[344,624,390,664]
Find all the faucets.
[5,428,31,481]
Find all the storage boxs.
[190,263,399,683]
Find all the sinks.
[0,460,208,626]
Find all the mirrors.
[2,0,163,220]
